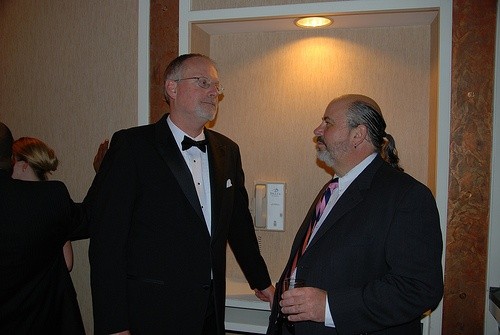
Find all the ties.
[283,179,337,291]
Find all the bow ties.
[181,135,207,153]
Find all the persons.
[0,121,109,335]
[88,53,276,335]
[267,94,445,335]
[11,137,74,273]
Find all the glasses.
[175,76,224,93]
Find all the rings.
[296,305,300,314]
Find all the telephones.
[255,183,285,233]
